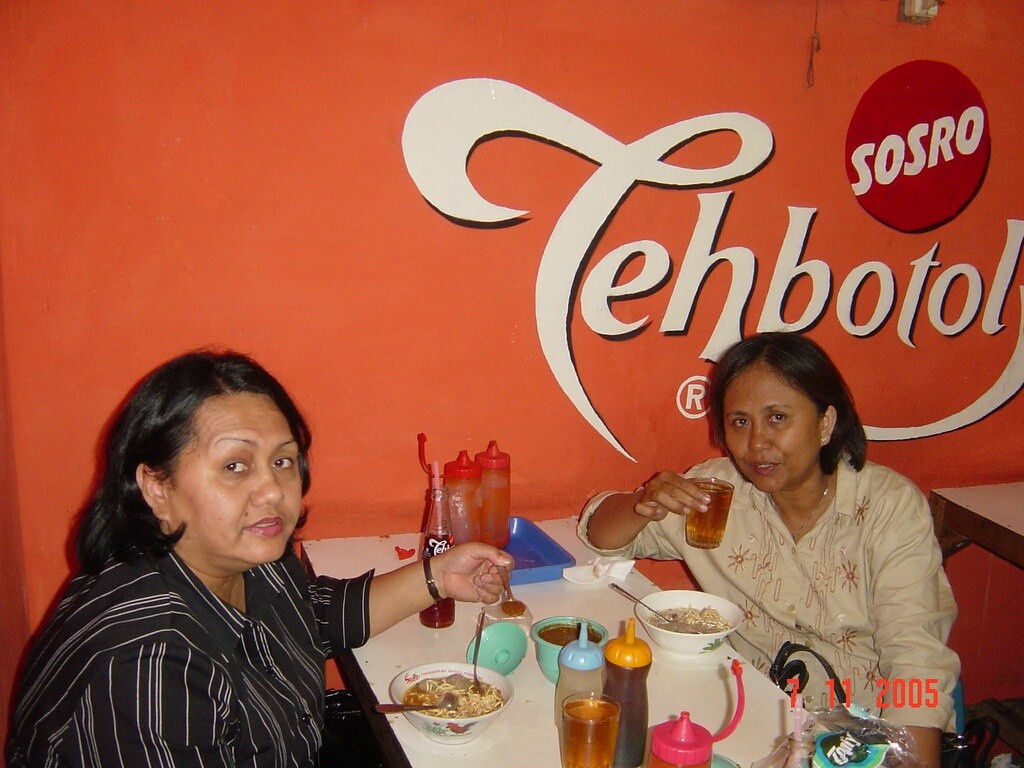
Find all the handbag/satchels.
[939,716,999,768]
[319,689,389,768]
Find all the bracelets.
[421,556,446,603]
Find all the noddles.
[400,674,505,720]
[646,603,732,634]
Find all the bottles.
[443,449,476,545]
[553,621,605,732]
[474,439,511,550]
[418,487,455,629]
[783,737,810,768]
[601,617,652,768]
[647,711,713,768]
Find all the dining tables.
[301,518,888,768]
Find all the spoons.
[374,692,457,714]
[495,564,526,616]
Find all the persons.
[5,347,515,768]
[574,332,962,768]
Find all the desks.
[927,482,1024,570]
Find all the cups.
[559,691,621,768]
[685,478,735,549]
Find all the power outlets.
[903,0,938,25]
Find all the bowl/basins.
[389,662,514,745]
[530,616,609,686]
[479,601,532,639]
[634,589,745,659]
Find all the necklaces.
[792,477,830,538]
